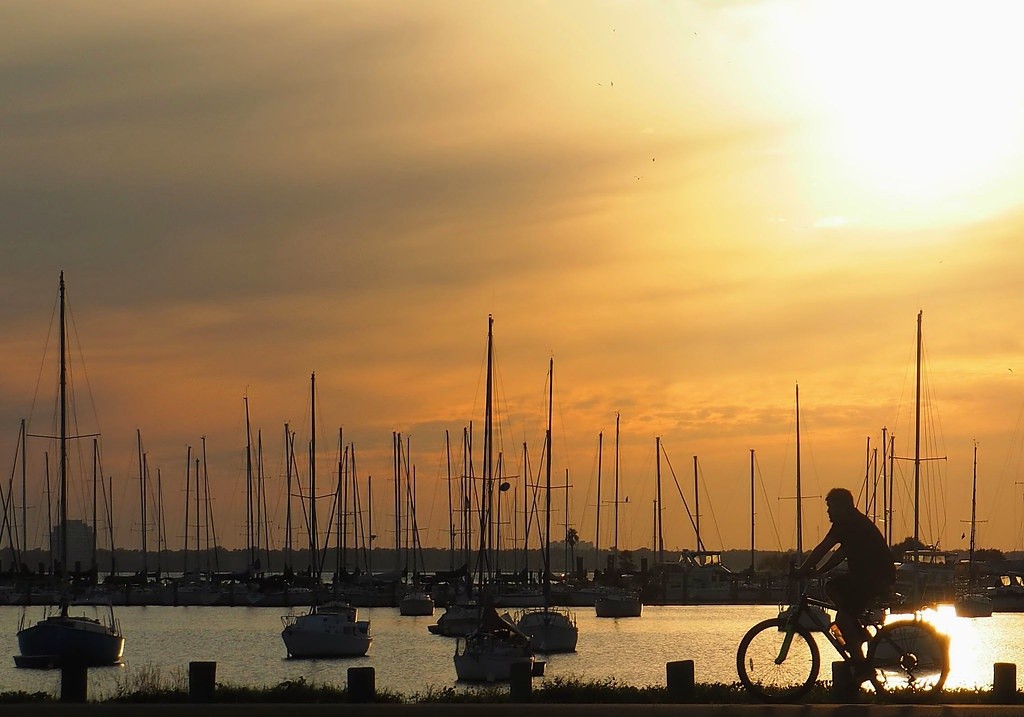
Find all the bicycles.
[735,567,950,693]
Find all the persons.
[792,488,897,681]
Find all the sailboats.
[0,267,1023,688]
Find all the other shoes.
[840,656,865,667]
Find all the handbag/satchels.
[780,604,829,630]
